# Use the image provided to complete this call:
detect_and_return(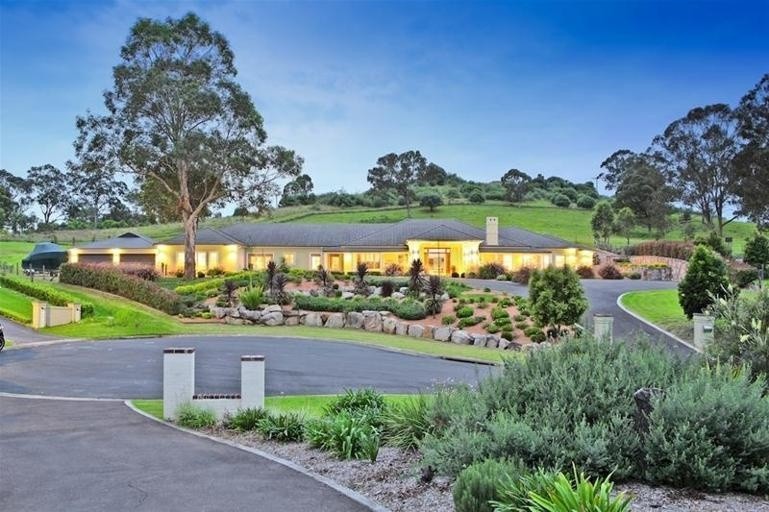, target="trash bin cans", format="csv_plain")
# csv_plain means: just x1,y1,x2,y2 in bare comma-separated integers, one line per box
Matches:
692,313,715,350
594,314,614,346
